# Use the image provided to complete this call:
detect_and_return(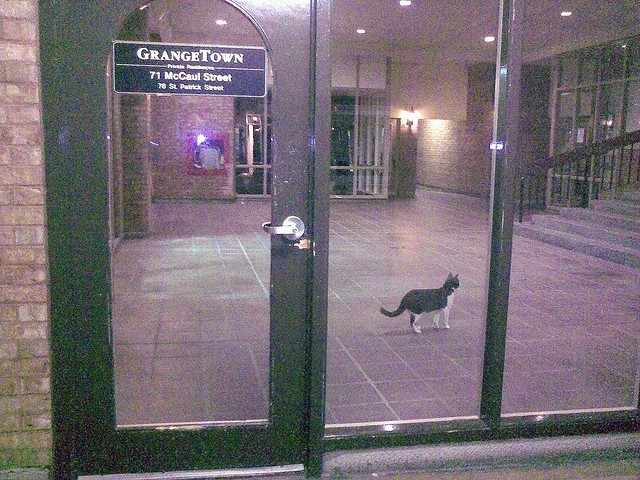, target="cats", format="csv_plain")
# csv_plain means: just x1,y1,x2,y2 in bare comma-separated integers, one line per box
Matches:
380,272,462,333
